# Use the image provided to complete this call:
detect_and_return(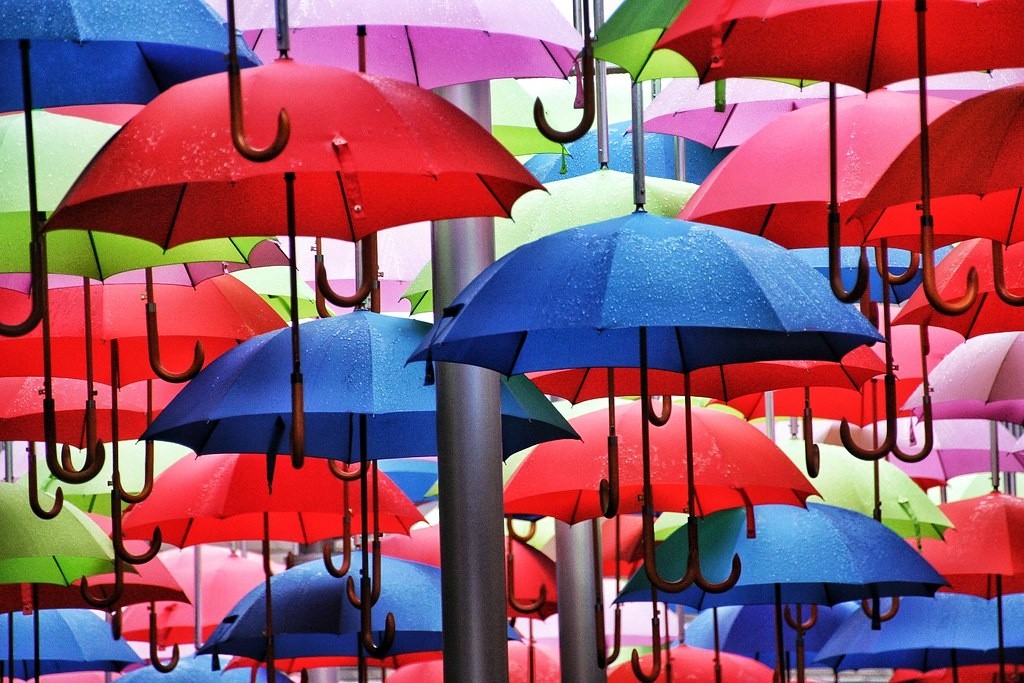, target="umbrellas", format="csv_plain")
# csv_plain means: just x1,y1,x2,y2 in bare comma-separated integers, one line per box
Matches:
0,0,1024,682
